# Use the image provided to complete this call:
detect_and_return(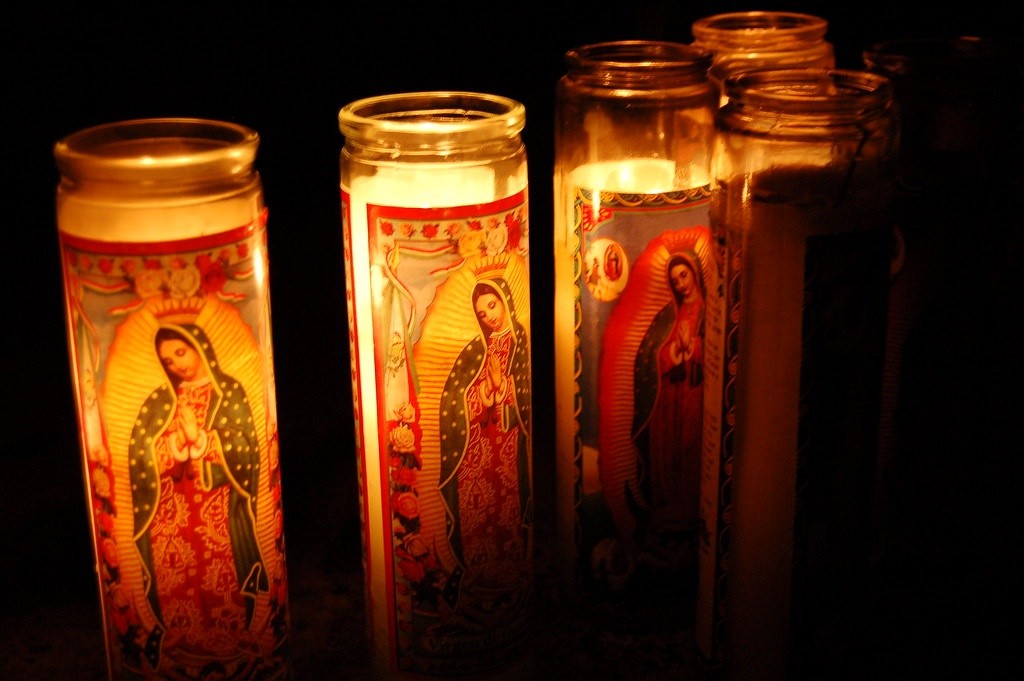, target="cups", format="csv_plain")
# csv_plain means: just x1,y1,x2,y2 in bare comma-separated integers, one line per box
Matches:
686,11,836,68
552,38,724,681
53,118,290,681
338,89,536,681
716,67,900,681
